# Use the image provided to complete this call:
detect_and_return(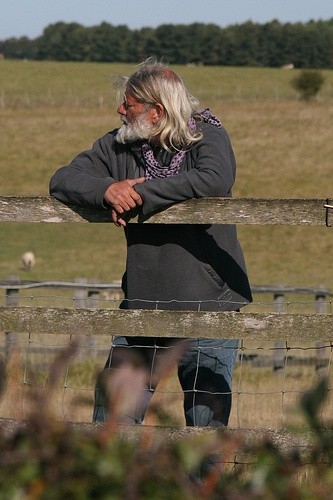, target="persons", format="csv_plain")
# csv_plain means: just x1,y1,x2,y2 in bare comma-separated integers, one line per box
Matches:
48,63,253,430
